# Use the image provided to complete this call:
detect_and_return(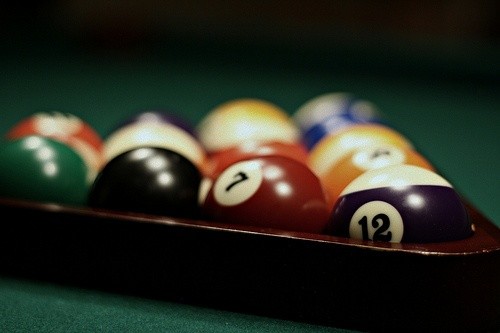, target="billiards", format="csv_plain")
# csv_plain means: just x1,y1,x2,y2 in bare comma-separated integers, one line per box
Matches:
20,89,437,202
1,135,93,202
331,164,475,242
89,145,208,214
202,155,330,232
